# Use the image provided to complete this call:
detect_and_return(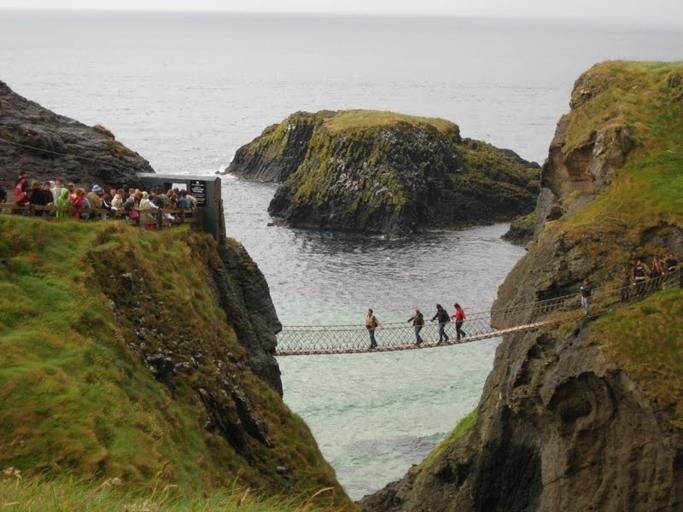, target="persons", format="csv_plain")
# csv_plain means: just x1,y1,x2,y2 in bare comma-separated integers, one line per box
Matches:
407,309,424,346
430,304,451,345
366,308,380,350
620,253,678,303
0,169,197,230
580,278,591,317
451,304,466,341
